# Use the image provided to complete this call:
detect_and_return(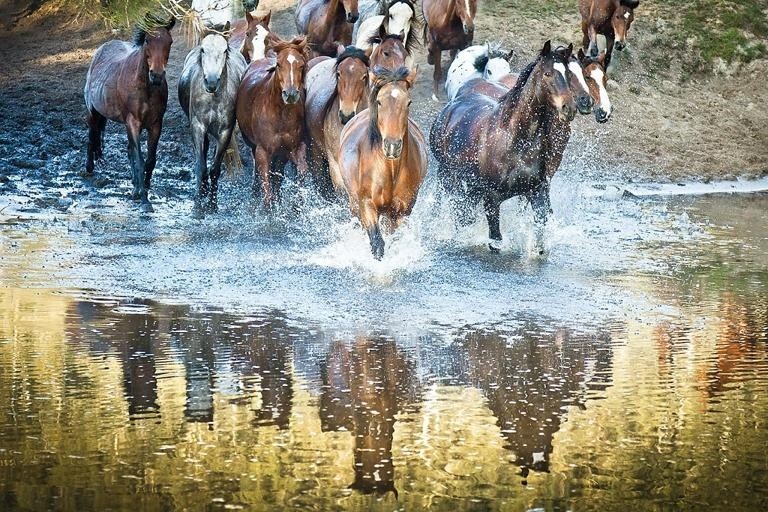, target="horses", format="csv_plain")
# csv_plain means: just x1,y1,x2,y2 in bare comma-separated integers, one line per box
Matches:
429,36,613,256
421,2,478,102
178,0,420,257
578,1,638,83
83,11,176,214
